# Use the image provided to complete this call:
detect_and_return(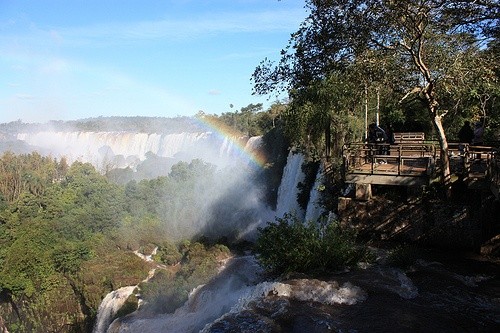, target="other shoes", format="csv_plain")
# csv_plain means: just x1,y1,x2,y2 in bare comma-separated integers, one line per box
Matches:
381,162,387,165
